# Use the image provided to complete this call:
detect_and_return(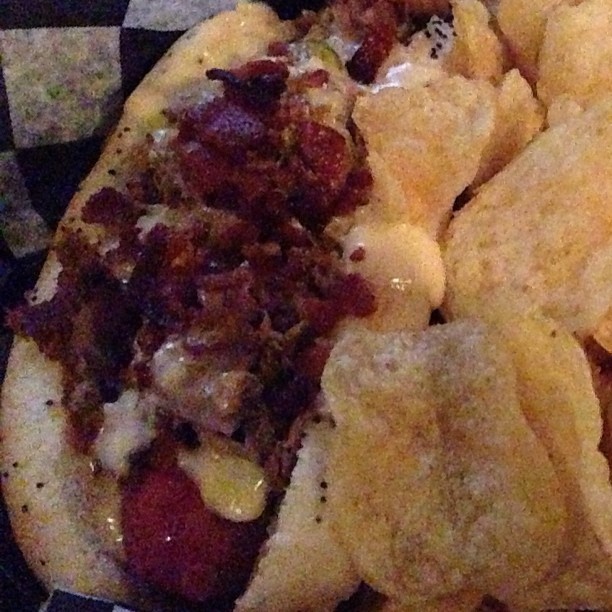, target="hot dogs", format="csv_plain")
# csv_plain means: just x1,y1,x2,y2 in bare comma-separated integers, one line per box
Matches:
0,0,491,611
